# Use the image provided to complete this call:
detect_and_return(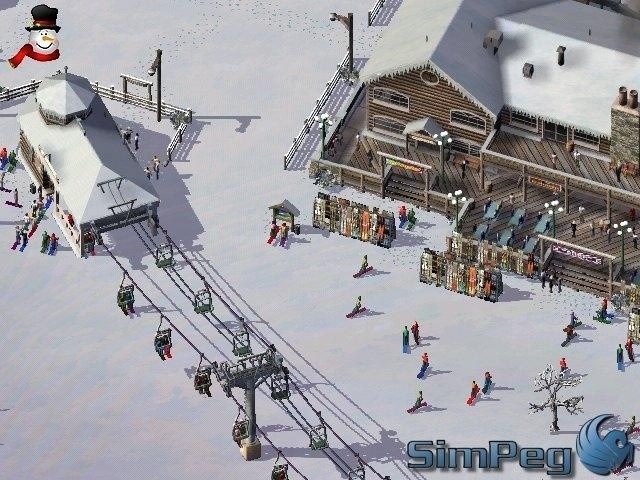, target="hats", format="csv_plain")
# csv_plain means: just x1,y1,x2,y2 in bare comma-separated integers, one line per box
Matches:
25,3,61,33
